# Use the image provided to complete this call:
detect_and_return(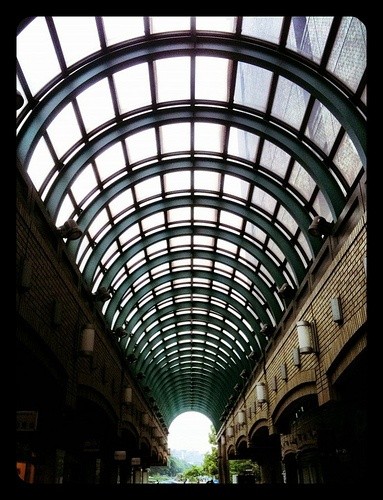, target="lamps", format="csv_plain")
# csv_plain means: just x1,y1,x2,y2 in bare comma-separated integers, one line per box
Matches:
102,364,110,385
58,219,82,241
78,323,95,358
281,363,288,381
51,300,62,330
292,347,301,369
271,374,278,393
260,324,275,336
296,319,318,354
248,351,261,361
93,287,110,303
330,297,343,325
308,216,333,238
277,283,295,299
90,351,97,370
17,253,33,295
126,353,137,366
114,327,127,339
121,369,269,456
